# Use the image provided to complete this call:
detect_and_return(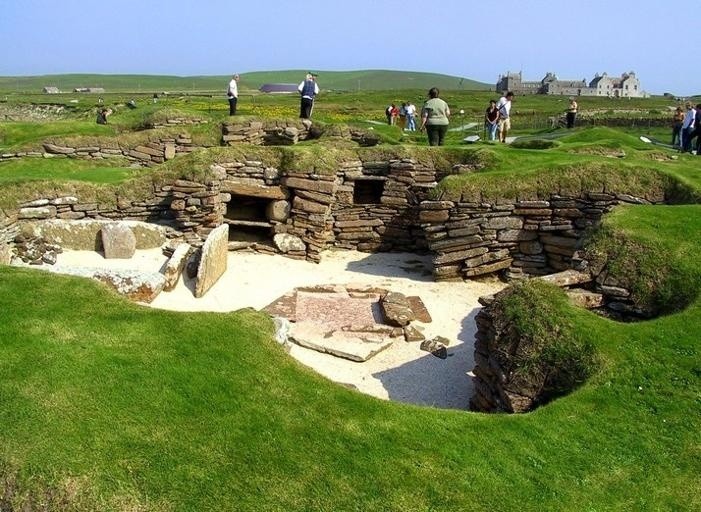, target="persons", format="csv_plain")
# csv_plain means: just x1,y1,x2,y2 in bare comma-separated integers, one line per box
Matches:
226,74,239,115
96,109,108,125
669,107,685,145
679,101,697,147
310,74,318,116
419,87,451,146
386,100,426,132
680,104,701,155
566,96,578,128
485,100,500,140
297,73,320,119
496,92,514,142
